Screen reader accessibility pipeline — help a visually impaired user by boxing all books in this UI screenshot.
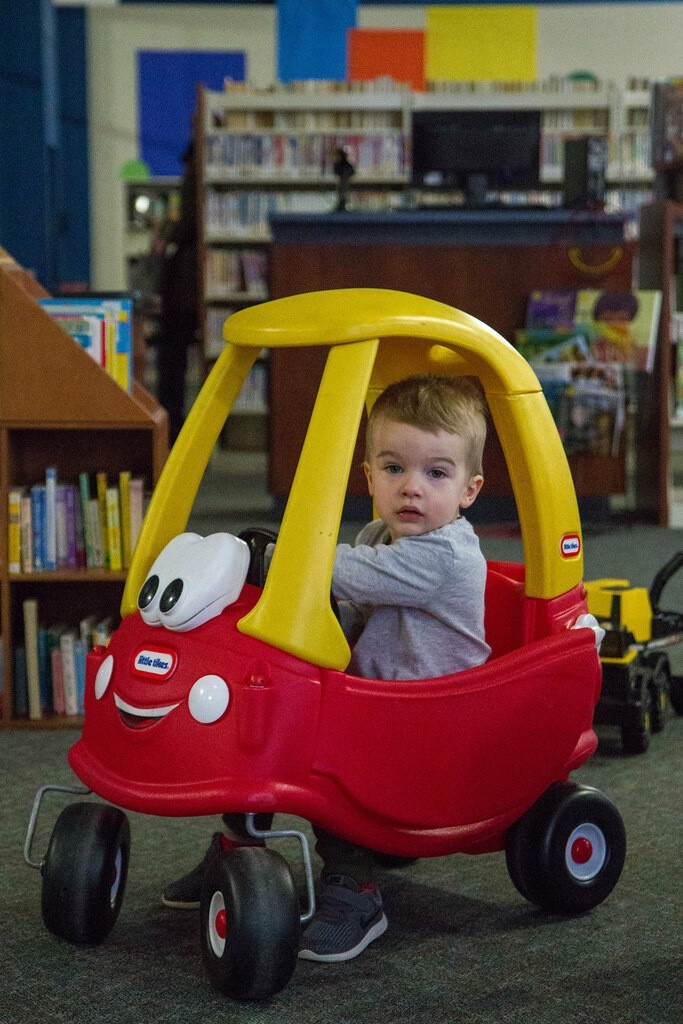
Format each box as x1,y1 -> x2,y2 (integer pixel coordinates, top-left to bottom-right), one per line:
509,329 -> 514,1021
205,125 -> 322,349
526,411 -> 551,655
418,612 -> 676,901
203,76 -> 652,413
38,296 -> 132,396
7,461 -> 151,575
11,597 -> 120,721
512,284 -> 664,458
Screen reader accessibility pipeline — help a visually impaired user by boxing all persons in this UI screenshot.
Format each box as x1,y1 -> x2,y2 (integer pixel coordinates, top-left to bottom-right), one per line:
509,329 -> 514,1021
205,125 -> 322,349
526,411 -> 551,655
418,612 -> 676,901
152,141 -> 201,449
159,374 -> 493,960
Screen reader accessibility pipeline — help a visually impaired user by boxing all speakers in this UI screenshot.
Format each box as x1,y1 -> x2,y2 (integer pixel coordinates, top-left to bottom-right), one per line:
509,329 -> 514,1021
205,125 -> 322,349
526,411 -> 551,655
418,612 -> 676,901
562,134 -> 610,211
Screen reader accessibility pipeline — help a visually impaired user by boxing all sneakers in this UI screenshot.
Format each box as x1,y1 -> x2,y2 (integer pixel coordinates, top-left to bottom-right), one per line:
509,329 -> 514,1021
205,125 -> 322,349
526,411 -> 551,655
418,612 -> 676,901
160,831 -> 271,910
295,863 -> 390,962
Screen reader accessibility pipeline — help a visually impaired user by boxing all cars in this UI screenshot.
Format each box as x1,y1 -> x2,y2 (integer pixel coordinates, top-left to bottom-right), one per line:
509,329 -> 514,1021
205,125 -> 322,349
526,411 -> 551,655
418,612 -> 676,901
37,289 -> 629,1009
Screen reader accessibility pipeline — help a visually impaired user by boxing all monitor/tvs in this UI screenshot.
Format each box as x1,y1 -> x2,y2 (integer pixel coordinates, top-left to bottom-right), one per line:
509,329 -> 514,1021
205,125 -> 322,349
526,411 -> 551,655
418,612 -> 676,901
409,110 -> 540,208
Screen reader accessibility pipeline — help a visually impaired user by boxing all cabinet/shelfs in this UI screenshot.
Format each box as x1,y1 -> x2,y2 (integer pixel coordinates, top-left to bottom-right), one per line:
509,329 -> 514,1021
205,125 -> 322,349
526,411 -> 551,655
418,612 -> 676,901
0,248 -> 168,730
195,82 -> 683,529
